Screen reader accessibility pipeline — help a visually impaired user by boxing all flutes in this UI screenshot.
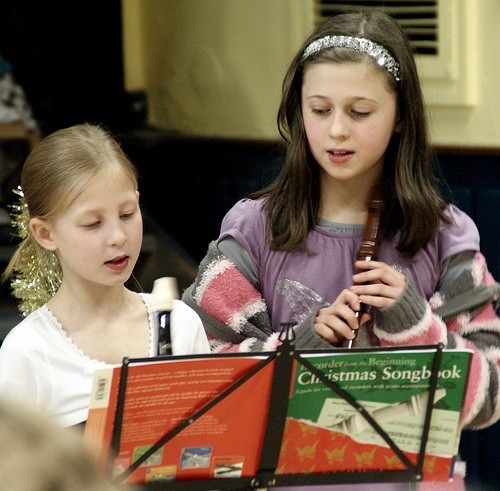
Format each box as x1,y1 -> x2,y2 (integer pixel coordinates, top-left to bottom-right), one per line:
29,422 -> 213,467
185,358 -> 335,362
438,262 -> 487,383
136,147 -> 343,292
340,182 -> 390,350
151,276 -> 182,356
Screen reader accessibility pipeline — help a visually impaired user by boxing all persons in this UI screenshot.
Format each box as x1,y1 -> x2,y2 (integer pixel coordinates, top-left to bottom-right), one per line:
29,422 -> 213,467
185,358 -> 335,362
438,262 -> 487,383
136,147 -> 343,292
1,125 -> 212,447
0,65 -> 43,242
182,10 -> 500,480
